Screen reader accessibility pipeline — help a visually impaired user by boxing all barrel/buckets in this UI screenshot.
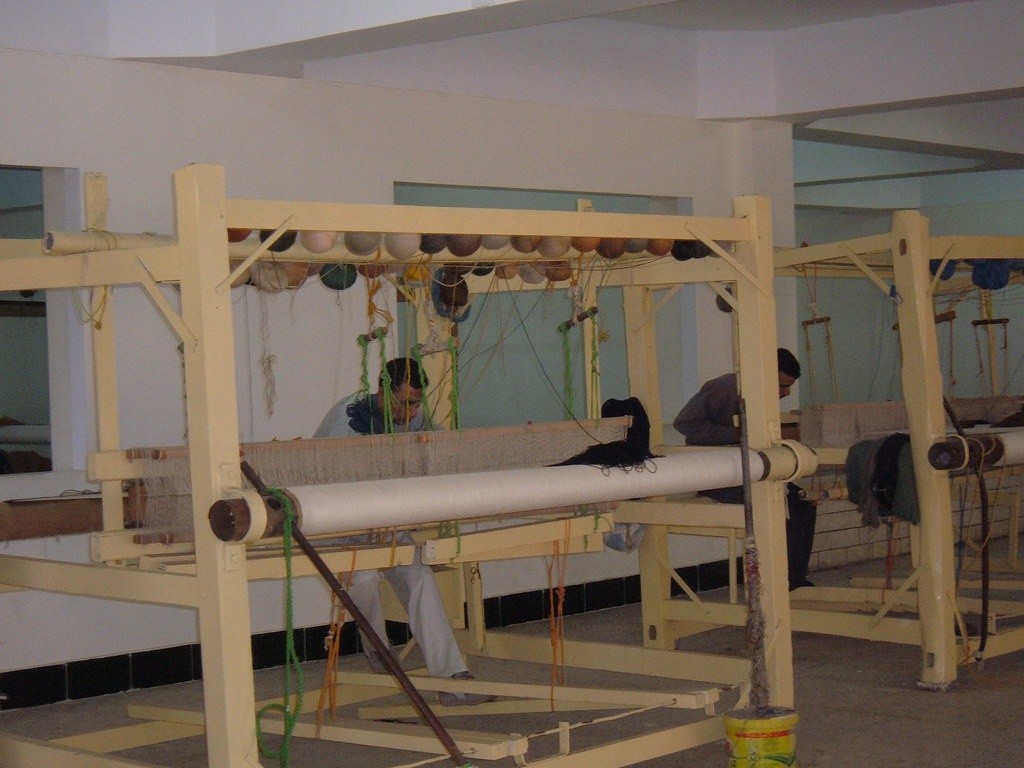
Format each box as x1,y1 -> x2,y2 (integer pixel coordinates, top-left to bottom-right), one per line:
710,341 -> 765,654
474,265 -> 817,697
723,703 -> 801,768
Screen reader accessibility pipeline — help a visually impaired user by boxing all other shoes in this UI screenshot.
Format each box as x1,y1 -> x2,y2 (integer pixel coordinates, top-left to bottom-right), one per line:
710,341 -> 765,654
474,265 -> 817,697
789,577 -> 815,592
361,638 -> 399,675
437,671 -> 498,706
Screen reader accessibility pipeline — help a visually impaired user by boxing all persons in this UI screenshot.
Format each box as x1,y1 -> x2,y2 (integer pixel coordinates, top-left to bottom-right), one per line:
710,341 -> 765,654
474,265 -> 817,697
311,358 -> 496,705
674,348 -> 815,590
